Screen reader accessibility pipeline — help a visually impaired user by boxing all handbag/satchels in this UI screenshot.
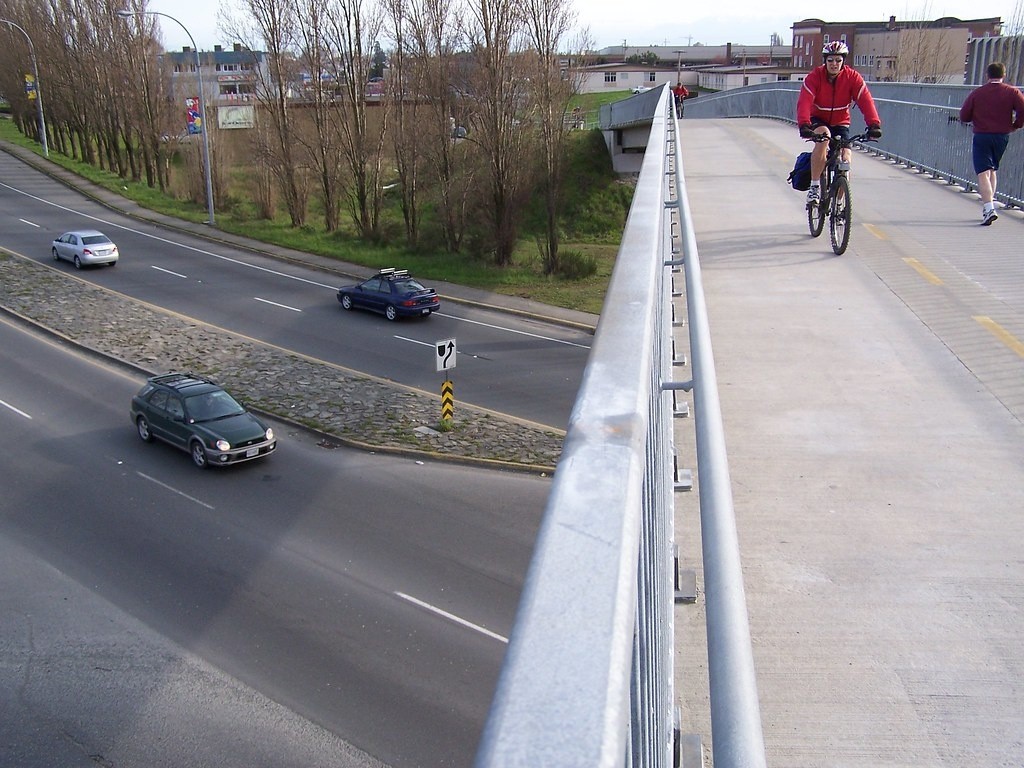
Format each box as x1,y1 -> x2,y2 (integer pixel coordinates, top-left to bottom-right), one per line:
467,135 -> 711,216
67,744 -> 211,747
787,153 -> 815,191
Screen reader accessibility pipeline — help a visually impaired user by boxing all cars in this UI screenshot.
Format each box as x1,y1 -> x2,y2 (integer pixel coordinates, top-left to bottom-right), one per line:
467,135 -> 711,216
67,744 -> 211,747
52,229 -> 119,270
129,372 -> 278,470
336,267 -> 440,321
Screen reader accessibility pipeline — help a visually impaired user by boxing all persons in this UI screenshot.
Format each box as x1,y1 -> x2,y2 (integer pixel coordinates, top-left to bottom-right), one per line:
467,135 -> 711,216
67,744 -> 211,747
206,397 -> 213,406
960,62 -> 1024,226
674,82 -> 689,117
795,40 -> 882,226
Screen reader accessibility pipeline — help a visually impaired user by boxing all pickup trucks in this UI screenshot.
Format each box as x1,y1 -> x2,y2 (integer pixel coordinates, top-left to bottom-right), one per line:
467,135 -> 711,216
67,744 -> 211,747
629,85 -> 655,94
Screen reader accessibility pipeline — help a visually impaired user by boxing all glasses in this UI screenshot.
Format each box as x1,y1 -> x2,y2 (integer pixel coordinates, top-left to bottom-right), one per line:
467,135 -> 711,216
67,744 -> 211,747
826,57 -> 845,62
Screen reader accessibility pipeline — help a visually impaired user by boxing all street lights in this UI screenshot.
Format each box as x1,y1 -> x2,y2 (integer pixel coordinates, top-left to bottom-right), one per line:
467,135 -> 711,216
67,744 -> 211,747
116,10 -> 216,225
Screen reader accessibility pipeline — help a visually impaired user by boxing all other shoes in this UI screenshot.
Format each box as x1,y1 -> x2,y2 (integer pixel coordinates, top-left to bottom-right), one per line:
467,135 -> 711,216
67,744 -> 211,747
981,209 -> 998,225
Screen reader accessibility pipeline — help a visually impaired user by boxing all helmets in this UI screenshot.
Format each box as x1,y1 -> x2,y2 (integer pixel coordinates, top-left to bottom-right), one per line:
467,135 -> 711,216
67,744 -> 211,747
822,40 -> 849,57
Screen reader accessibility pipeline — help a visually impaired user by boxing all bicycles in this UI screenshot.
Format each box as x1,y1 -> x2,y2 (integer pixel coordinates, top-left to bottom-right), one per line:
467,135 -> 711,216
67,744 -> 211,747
800,133 -> 882,255
675,95 -> 687,119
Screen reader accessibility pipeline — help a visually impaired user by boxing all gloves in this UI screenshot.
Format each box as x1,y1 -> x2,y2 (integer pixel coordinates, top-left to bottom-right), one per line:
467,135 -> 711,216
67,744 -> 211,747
800,127 -> 813,138
867,129 -> 881,138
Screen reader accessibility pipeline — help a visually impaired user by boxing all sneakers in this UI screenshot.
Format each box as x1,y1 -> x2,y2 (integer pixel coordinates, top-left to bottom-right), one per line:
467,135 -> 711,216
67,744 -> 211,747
836,208 -> 844,226
807,185 -> 820,204
